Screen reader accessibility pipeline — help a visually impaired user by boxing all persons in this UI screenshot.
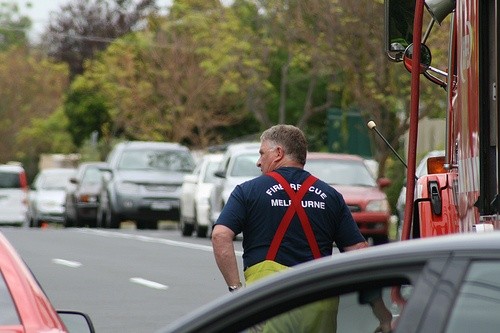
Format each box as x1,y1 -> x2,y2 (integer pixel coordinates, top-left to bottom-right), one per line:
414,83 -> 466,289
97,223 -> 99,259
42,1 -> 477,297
210,124 -> 393,333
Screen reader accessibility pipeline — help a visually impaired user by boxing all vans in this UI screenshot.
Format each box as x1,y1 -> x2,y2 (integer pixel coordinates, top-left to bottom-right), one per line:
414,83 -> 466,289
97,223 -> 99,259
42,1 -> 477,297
0,164 -> 27,226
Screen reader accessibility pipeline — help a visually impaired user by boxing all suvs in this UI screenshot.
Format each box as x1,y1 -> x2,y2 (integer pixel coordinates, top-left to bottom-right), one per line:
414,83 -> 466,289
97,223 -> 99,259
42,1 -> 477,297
97,142 -> 196,228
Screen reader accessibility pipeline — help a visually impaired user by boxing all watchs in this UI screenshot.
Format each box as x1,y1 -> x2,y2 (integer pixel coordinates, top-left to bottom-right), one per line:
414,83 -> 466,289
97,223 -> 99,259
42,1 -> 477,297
228,282 -> 242,292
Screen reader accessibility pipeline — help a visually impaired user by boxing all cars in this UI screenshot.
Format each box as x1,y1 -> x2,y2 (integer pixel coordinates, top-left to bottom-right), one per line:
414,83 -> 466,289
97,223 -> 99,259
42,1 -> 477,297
302,152 -> 392,246
62,161 -> 106,227
26,168 -> 74,229
0,230 -> 95,333
173,151 -> 226,238
207,142 -> 265,240
